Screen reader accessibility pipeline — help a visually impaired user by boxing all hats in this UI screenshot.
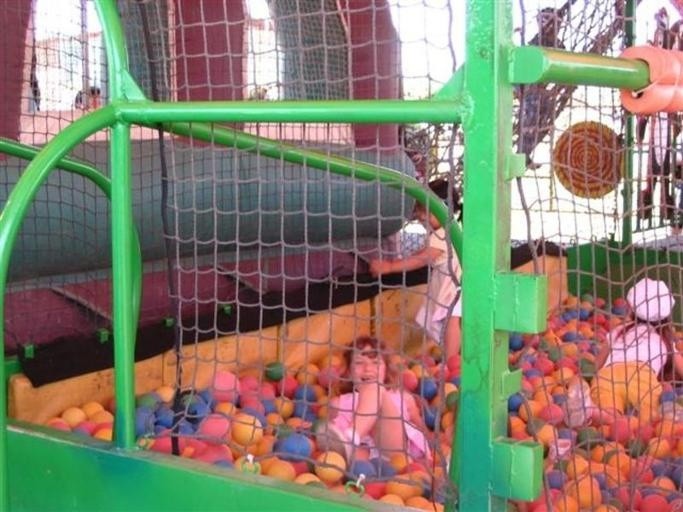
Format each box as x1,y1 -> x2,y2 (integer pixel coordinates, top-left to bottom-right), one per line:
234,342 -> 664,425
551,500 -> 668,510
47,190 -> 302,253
627,278 -> 676,323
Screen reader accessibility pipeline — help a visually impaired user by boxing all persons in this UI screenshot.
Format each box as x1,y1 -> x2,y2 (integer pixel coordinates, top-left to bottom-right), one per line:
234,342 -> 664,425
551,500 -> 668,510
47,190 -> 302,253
369,181 -> 462,370
315,335 -> 432,484
514,9 -> 567,168
590,277 -> 683,422
76,87 -> 100,108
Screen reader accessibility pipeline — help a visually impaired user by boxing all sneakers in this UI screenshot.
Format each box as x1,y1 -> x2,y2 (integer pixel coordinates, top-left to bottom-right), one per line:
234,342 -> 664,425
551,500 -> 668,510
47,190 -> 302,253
317,425 -> 349,465
